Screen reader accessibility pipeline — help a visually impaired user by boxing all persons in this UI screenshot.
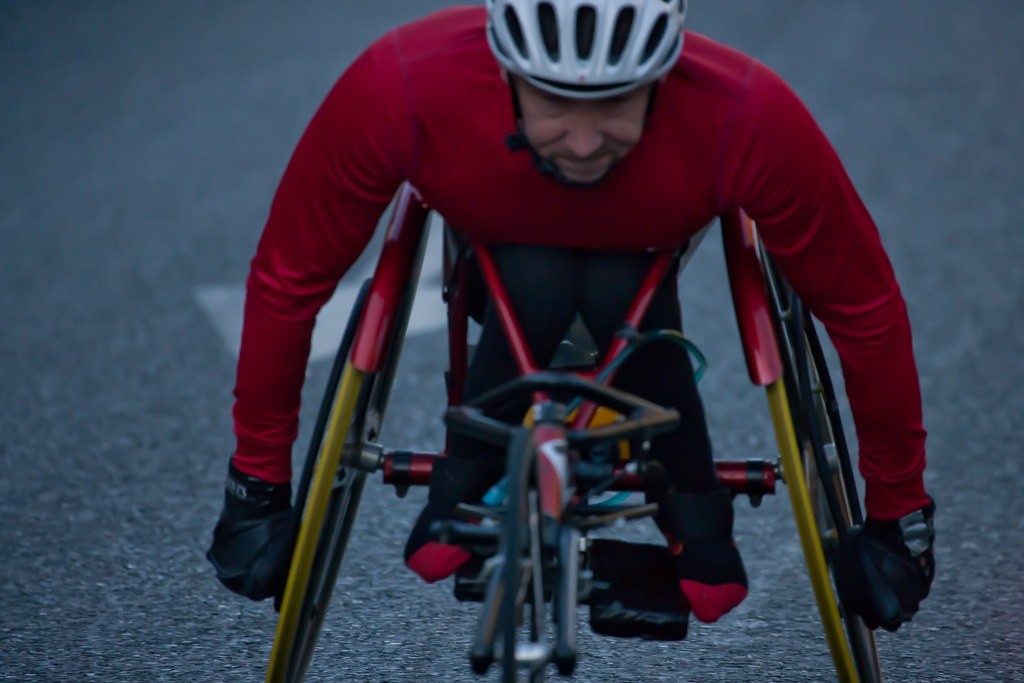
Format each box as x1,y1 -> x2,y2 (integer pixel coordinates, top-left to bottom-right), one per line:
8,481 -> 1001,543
205,0 -> 938,633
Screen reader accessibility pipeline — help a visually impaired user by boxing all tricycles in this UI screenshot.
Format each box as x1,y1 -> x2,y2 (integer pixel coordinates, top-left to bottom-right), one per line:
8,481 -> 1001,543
265,174 -> 881,683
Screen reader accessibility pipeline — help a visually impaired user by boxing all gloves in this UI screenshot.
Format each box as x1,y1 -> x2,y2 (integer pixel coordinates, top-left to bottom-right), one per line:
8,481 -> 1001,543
205,453 -> 296,612
839,493 -> 936,633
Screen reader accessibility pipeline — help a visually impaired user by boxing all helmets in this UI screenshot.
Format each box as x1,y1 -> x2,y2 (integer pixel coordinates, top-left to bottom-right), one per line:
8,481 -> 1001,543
485,0 -> 688,99
